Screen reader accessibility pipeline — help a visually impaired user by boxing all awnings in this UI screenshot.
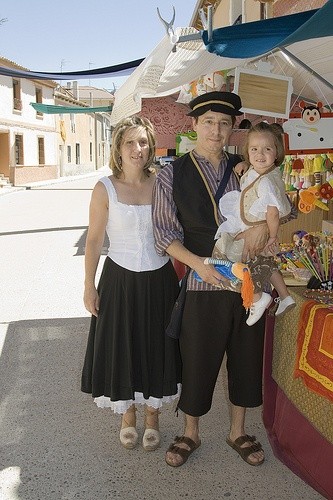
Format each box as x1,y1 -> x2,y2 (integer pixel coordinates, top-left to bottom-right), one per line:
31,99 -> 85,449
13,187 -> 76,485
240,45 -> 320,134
109,0 -> 332,128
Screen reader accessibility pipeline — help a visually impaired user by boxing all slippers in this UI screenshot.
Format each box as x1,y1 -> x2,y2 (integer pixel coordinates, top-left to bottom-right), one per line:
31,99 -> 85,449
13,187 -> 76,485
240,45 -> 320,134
166,436 -> 202,467
226,434 -> 266,466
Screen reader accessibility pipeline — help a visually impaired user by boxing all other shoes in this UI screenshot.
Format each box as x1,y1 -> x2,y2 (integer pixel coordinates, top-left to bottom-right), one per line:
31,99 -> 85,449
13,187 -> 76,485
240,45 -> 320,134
274,295 -> 295,317
246,291 -> 273,326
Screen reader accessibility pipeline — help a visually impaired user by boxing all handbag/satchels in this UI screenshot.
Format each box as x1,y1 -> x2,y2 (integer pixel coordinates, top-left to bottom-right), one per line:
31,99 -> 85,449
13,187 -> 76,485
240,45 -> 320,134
166,272 -> 187,341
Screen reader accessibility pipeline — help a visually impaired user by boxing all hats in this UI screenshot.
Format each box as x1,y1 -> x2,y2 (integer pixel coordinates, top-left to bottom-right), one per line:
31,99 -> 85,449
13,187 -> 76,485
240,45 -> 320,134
185,90 -> 245,117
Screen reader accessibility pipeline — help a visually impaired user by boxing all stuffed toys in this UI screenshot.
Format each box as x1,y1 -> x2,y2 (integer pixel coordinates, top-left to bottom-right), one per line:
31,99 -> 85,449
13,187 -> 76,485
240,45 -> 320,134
192,257 -> 256,309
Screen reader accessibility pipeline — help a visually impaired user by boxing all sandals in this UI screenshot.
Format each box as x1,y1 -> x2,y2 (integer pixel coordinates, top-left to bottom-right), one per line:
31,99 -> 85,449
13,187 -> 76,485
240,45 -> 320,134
143,410 -> 161,450
120,412 -> 138,448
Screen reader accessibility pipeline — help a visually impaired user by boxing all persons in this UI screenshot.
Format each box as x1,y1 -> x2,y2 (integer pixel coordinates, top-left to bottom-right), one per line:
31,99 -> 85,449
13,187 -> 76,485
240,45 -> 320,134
82,114 -> 184,453
149,89 -> 272,468
208,120 -> 297,328
